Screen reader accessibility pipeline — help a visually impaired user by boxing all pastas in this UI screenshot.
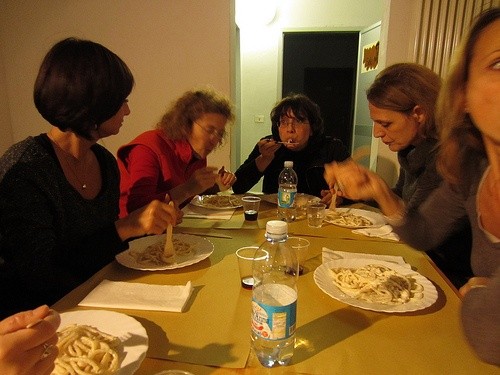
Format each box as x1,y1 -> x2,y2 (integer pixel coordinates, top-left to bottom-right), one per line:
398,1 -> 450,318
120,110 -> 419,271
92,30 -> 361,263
51,322 -> 123,375
327,264 -> 424,306
129,237 -> 192,264
324,212 -> 371,227
201,195 -> 243,207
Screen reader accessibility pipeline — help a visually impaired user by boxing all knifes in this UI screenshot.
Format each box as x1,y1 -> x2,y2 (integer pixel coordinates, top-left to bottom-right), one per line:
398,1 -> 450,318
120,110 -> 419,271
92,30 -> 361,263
177,232 -> 233,239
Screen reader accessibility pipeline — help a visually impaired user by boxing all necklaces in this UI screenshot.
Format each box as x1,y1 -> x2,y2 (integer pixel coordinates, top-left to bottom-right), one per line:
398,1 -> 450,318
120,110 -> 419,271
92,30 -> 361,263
56,139 -> 87,189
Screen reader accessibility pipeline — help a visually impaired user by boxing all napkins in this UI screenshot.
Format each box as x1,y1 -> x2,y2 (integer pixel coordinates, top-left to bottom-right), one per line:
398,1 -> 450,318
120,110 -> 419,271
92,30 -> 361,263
352,225 -> 401,242
322,246 -> 412,270
77,279 -> 194,312
182,206 -> 236,219
176,219 -> 216,228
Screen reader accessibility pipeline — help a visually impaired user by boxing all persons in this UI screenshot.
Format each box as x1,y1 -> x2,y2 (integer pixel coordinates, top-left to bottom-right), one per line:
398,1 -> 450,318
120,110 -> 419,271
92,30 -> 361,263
324,6 -> 500,366
0,38 -> 184,375
234,94 -> 352,209
115,87 -> 236,230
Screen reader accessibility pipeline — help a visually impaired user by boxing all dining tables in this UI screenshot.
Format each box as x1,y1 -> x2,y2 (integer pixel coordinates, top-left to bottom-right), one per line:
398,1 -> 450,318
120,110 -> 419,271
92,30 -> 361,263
51,194 -> 500,375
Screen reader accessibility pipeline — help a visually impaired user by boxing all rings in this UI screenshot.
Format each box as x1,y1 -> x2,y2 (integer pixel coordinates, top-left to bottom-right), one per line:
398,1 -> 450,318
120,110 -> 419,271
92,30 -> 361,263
41,343 -> 54,358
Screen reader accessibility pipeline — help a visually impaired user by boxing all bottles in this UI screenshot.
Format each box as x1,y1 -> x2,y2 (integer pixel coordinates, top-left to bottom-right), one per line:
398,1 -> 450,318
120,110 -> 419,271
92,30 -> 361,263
277,161 -> 298,224
251,220 -> 299,369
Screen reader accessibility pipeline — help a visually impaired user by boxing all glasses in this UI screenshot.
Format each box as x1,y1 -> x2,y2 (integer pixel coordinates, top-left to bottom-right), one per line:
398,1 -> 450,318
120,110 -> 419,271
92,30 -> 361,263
193,121 -> 226,140
276,115 -> 305,128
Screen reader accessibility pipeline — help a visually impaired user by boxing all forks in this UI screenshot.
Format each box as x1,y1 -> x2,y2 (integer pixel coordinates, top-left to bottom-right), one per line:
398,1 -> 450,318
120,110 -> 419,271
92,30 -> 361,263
164,200 -> 174,257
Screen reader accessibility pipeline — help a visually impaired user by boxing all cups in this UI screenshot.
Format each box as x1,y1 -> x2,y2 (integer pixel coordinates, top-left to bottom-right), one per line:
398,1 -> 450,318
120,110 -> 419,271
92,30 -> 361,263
241,196 -> 261,221
306,203 -> 327,229
235,247 -> 269,290
285,237 -> 310,276
154,370 -> 194,375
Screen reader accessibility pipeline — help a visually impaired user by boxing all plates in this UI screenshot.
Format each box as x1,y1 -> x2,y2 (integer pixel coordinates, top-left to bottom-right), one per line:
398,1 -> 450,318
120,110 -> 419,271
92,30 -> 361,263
115,234 -> 213,271
49,310 -> 149,375
312,259 -> 438,313
322,209 -> 388,228
190,195 -> 244,209
263,193 -> 321,206
